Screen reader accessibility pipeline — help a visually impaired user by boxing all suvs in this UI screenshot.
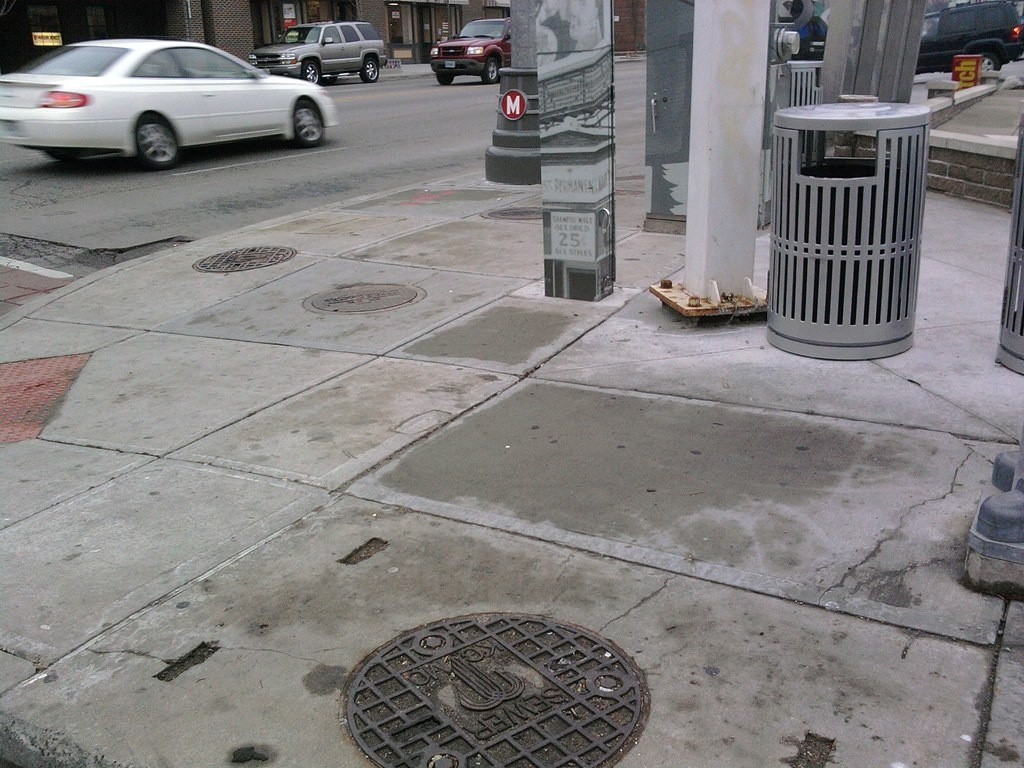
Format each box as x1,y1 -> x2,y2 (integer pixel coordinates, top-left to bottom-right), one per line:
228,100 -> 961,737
247,22 -> 390,85
428,16 -> 512,86
913,1 -> 1024,77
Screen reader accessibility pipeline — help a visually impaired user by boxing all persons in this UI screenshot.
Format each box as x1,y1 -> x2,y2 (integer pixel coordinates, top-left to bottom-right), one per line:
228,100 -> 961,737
790,0 -> 828,59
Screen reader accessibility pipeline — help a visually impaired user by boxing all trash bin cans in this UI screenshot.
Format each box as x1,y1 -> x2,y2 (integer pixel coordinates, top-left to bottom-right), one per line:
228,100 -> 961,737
787,61 -> 820,163
996,111 -> 1024,376
767,101 -> 929,360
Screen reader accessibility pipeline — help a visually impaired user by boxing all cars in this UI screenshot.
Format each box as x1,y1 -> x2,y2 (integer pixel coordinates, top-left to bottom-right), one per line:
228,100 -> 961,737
0,37 -> 341,174
